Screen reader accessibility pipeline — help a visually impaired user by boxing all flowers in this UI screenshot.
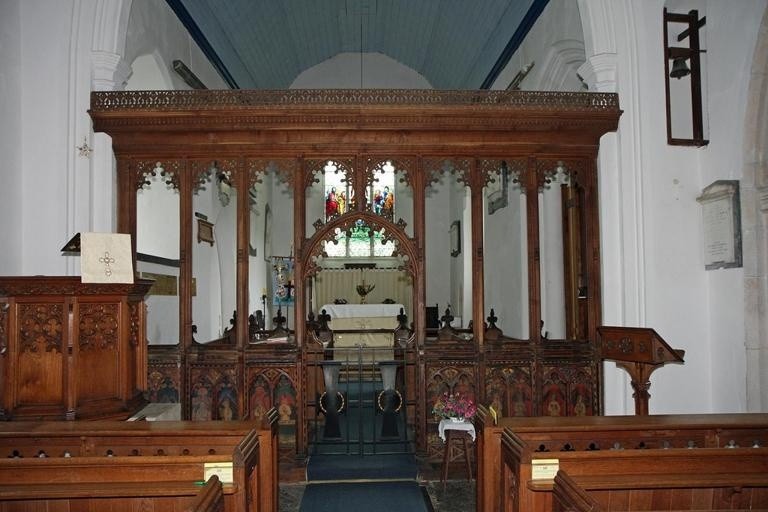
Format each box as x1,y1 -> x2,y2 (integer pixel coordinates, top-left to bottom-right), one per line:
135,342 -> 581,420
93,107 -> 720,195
432,391 -> 477,419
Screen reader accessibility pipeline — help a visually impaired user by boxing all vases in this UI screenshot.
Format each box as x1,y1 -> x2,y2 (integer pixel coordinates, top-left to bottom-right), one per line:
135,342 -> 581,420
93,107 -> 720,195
448,413 -> 466,422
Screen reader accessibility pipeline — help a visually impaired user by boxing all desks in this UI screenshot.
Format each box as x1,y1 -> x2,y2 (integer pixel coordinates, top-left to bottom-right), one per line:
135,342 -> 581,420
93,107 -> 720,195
438,419 -> 477,486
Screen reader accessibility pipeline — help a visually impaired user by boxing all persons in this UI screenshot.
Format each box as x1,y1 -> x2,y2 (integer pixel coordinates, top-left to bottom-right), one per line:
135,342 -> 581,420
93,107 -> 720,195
327,186 -> 393,226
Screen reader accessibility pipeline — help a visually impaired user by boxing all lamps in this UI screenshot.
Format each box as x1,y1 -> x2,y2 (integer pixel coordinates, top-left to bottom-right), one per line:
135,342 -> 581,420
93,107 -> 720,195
661,7 -> 709,149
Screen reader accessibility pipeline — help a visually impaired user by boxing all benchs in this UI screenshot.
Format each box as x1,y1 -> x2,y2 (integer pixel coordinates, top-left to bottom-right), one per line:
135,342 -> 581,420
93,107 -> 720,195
476,411 -> 731,509
0,405 -> 280,511
